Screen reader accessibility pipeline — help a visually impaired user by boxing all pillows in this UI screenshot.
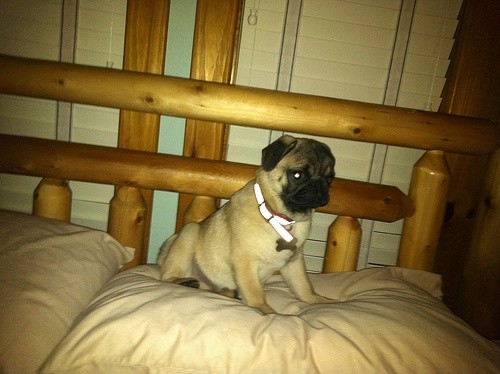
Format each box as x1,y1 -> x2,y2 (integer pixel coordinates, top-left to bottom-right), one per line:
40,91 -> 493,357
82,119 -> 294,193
0,208 -> 136,374
36,264 -> 500,374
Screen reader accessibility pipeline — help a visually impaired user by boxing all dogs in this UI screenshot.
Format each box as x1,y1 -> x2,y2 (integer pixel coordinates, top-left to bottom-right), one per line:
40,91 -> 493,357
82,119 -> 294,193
155,134 -> 338,319
